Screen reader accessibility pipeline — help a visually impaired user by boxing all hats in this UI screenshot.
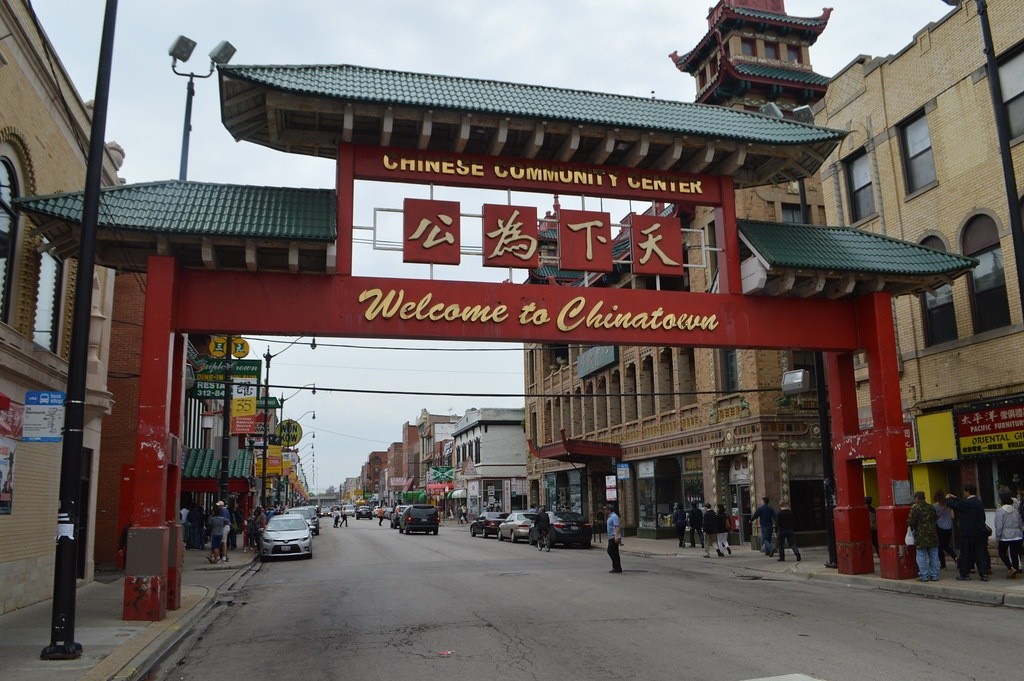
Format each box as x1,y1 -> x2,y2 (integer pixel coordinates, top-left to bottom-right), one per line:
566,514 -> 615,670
604,504 -> 614,510
216,500 -> 224,506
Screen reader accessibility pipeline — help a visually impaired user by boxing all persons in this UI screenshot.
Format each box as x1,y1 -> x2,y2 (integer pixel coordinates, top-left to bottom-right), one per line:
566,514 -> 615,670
533,506 -> 551,541
334,509 -> 339,528
906,492 -> 940,581
557,502 -> 571,512
996,479 -> 1024,580
486,505 -> 502,512
457,503 -> 468,524
866,496 -> 879,557
931,489 -> 960,569
339,511 -> 347,527
118,523 -> 131,570
672,501 -> 686,548
378,505 -> 385,526
689,500 -> 732,558
602,504 -> 622,573
749,496 -> 776,558
206,500 -> 243,563
945,485 -> 991,582
180,501 -> 205,551
671,501 -> 679,514
529,502 -> 538,513
250,500 -> 291,551
774,497 -> 802,561
448,506 -> 456,522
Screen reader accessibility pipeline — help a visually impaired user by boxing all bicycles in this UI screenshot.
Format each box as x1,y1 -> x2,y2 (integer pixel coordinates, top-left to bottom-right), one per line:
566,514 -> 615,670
536,528 -> 551,552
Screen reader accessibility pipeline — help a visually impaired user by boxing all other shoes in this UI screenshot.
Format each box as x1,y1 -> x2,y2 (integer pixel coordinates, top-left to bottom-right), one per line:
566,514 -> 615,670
1014,573 -> 1019,579
679,540 -> 685,547
716,549 -> 724,557
609,568 -> 622,573
689,544 -> 695,547
766,550 -> 774,557
702,542 -> 704,548
778,558 -> 785,561
1006,567 -> 1016,578
703,555 -> 710,558
955,556 -> 961,569
797,553 -> 801,561
939,566 -> 947,571
956,574 -> 971,580
981,574 -> 988,580
726,546 -> 731,554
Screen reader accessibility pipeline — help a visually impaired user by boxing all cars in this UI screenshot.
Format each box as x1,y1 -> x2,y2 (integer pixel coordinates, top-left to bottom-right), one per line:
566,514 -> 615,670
319,504 -> 332,516
497,512 -> 538,542
356,506 -> 372,520
470,512 -> 509,538
342,504 -> 355,517
372,506 -> 394,519
282,505 -> 320,535
528,511 -> 592,548
259,514 -> 317,560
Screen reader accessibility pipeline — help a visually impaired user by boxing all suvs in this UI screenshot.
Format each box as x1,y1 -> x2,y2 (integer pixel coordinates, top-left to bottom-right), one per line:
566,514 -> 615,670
398,504 -> 439,535
390,505 -> 409,529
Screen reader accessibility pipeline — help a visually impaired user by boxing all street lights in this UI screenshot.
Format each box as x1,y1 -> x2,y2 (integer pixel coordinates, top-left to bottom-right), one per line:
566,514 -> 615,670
291,432 -> 315,507
277,382 -> 316,507
169,35 -> 236,182
285,410 -> 315,507
261,336 -> 317,508
757,101 -> 840,568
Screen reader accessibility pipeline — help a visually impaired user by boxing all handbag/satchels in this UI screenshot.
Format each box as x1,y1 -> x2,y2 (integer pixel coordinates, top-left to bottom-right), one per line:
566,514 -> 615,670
985,524 -> 992,536
905,526 -> 914,545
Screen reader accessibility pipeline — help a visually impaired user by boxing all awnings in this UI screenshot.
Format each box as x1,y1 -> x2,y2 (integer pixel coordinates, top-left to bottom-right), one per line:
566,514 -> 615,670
446,489 -> 466,498
402,490 -> 427,500
